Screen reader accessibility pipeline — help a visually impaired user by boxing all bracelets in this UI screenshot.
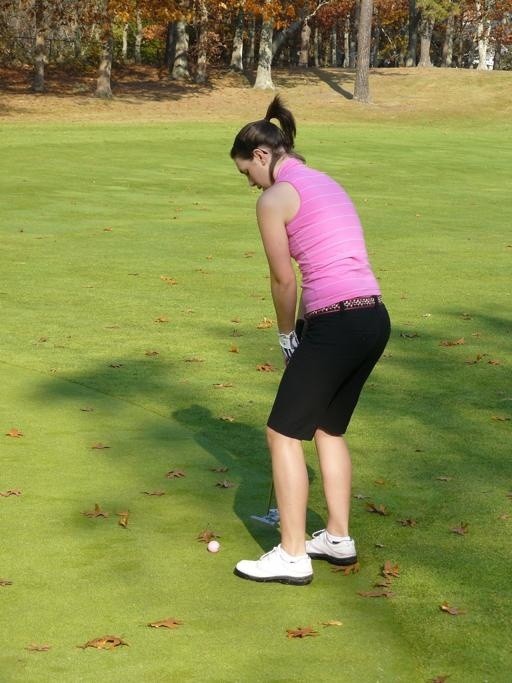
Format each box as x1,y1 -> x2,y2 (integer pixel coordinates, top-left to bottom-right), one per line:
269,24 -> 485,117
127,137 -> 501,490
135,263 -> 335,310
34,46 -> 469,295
277,329 -> 299,359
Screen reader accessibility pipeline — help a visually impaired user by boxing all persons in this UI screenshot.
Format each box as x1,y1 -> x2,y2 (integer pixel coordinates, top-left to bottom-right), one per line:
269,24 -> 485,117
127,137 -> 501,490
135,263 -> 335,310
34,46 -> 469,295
229,95 -> 391,584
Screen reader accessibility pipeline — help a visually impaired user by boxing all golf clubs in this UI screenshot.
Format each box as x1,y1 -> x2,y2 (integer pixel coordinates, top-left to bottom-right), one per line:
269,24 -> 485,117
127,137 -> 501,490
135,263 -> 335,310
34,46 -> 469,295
251,317 -> 306,526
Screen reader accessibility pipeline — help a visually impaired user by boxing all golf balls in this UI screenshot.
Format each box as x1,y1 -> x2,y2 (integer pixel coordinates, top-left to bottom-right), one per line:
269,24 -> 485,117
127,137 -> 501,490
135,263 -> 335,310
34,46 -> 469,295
209,537 -> 223,556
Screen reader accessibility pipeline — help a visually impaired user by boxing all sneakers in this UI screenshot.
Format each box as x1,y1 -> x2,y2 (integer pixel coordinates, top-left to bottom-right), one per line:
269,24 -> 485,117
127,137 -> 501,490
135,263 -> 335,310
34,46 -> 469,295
235,543 -> 314,586
305,527 -> 358,567
